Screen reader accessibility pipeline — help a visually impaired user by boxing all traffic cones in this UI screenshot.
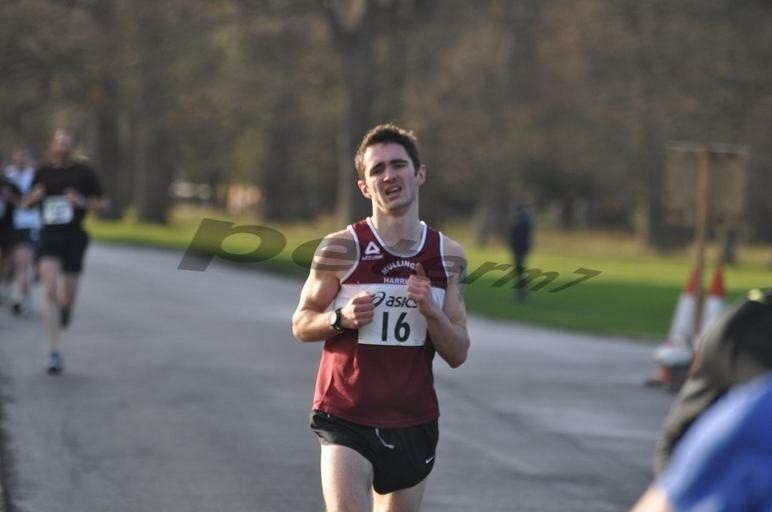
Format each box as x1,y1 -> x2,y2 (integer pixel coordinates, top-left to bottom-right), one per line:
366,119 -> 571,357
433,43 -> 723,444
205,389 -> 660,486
660,266 -> 724,380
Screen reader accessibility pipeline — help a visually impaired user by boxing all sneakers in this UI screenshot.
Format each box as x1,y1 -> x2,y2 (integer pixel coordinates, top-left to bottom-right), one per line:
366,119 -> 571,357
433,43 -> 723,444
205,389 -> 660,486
45,304 -> 70,374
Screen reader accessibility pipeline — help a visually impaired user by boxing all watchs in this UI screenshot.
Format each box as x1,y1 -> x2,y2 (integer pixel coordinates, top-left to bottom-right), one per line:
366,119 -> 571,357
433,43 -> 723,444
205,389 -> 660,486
329,308 -> 345,334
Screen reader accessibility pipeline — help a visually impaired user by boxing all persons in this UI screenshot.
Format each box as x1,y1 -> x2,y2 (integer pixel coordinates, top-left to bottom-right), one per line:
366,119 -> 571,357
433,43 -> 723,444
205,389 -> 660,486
620,285 -> 772,512
511,197 -> 535,294
0,128 -> 114,375
292,124 -> 469,512
